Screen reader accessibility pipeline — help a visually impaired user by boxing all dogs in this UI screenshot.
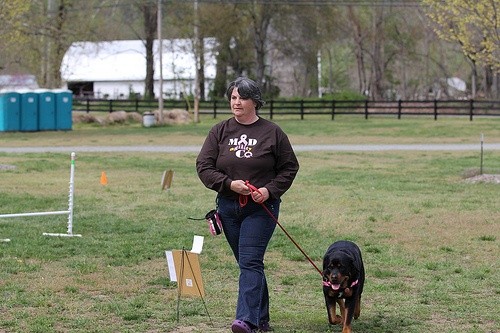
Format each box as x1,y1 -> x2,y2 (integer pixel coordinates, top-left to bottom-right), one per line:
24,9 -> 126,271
322,240 -> 365,333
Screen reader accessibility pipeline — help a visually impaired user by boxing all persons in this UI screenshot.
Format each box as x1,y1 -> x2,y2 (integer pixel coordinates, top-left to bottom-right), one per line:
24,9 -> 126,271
195,76 -> 301,333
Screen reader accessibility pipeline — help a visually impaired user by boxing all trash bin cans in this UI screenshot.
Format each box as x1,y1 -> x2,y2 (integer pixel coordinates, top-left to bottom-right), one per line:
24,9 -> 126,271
0,90 -> 20,133
36,89 -> 56,131
53,89 -> 73,132
19,89 -> 37,132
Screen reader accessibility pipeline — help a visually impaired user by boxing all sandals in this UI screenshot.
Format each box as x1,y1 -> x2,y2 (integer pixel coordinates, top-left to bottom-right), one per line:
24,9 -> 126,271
259,323 -> 273,331
231,320 -> 251,333
